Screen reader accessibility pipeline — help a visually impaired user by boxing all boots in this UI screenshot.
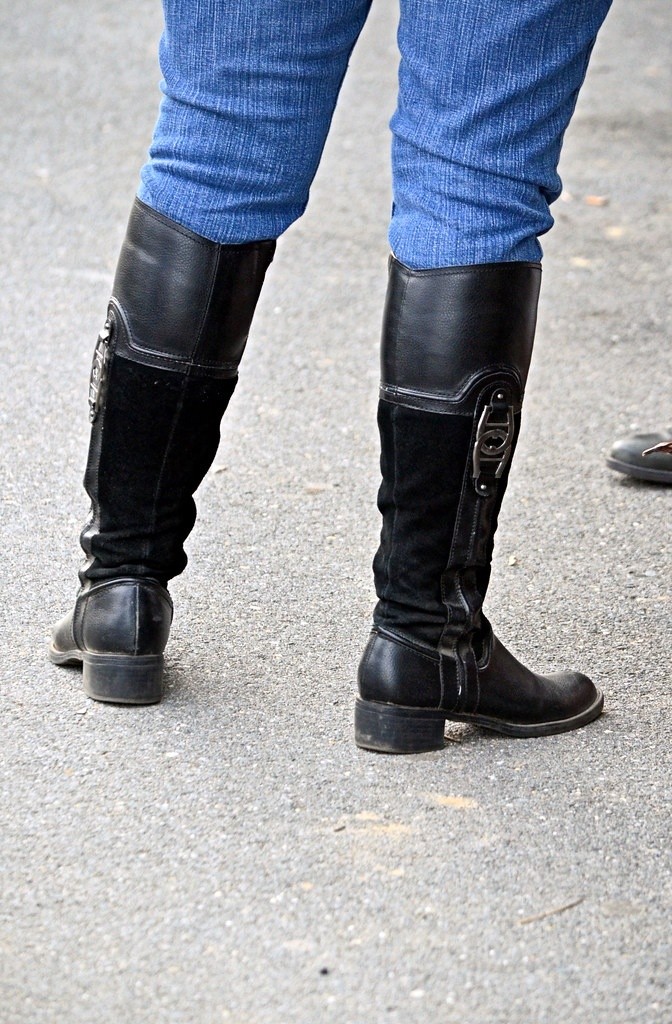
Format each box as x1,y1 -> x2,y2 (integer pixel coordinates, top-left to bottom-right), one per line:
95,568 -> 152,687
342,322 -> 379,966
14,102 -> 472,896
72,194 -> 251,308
354,251 -> 604,756
49,198 -> 277,706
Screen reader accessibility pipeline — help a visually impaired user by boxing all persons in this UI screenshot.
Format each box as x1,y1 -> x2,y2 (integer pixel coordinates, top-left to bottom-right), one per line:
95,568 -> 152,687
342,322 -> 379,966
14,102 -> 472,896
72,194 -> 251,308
49,0 -> 604,753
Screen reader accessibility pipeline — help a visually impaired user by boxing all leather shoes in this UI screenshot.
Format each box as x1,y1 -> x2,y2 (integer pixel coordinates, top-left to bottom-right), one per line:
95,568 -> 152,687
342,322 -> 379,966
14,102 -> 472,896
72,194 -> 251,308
606,431 -> 670,483
